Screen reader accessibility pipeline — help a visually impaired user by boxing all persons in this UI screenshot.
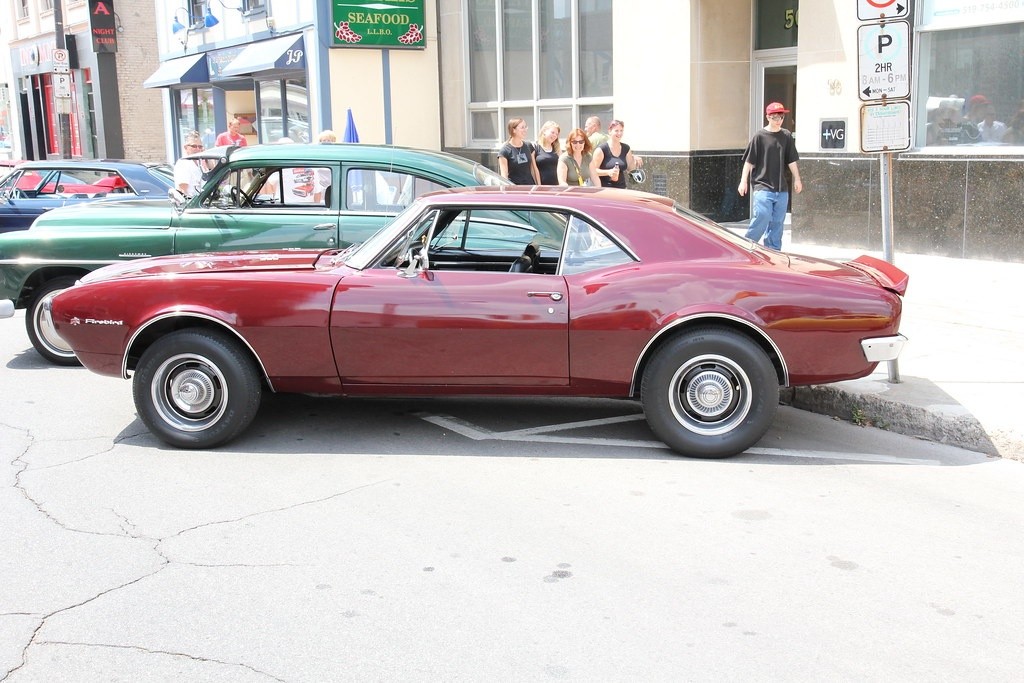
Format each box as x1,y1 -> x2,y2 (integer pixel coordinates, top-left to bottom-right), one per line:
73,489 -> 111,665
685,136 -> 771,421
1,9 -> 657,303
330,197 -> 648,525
555,128 -> 602,188
592,120 -> 643,190
582,116 -> 610,159
214,117 -> 248,189
173,130 -> 222,200
530,121 -> 563,186
258,137 -> 321,204
738,102 -> 802,251
926,92 -> 1024,147
312,129 -> 347,204
496,117 -> 538,185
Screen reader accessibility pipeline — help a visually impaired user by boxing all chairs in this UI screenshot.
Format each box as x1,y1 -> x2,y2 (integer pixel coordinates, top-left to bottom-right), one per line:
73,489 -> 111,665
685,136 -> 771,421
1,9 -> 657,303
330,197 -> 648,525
325,185 -> 353,208
508,242 -> 541,273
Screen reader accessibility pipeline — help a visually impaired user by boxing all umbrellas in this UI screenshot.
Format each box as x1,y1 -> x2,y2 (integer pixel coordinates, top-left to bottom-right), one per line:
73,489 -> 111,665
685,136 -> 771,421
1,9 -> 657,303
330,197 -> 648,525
341,106 -> 367,203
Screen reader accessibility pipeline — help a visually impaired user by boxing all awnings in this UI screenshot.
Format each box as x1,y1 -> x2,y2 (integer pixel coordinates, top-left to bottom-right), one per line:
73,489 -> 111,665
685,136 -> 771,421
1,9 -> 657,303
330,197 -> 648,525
221,32 -> 305,79
142,51 -> 210,89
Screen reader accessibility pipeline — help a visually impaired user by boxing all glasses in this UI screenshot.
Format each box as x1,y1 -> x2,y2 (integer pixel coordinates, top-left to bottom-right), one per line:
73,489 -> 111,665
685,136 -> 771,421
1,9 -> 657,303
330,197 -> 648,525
187,144 -> 203,149
516,126 -> 528,130
769,115 -> 784,121
570,139 -> 584,144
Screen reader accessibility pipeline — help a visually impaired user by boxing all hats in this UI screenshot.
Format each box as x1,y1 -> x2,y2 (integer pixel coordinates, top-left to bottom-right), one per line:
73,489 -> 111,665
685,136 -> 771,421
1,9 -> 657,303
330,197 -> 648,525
968,95 -> 995,110
766,102 -> 790,115
627,168 -> 646,185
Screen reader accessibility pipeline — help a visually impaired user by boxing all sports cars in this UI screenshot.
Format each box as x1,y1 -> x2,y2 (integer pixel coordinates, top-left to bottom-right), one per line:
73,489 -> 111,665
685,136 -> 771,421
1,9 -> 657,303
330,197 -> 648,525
47,183 -> 910,460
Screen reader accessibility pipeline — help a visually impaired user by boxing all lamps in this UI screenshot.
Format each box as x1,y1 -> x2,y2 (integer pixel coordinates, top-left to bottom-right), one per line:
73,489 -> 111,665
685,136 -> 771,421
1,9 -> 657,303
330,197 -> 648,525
173,7 -> 205,34
205,0 -> 241,27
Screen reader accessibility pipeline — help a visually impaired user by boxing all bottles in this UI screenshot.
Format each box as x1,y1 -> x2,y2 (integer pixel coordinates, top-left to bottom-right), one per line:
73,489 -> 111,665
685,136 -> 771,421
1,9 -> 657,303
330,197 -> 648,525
611,163 -> 619,181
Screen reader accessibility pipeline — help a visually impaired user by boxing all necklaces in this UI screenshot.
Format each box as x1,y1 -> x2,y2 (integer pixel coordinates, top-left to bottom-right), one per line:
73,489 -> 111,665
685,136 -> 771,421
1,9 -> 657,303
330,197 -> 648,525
513,143 -> 522,155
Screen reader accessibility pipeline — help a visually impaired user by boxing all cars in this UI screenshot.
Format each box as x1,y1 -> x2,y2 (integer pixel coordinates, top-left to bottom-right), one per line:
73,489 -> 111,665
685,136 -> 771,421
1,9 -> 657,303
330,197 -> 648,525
0,158 -> 275,237
0,140 -> 606,368
